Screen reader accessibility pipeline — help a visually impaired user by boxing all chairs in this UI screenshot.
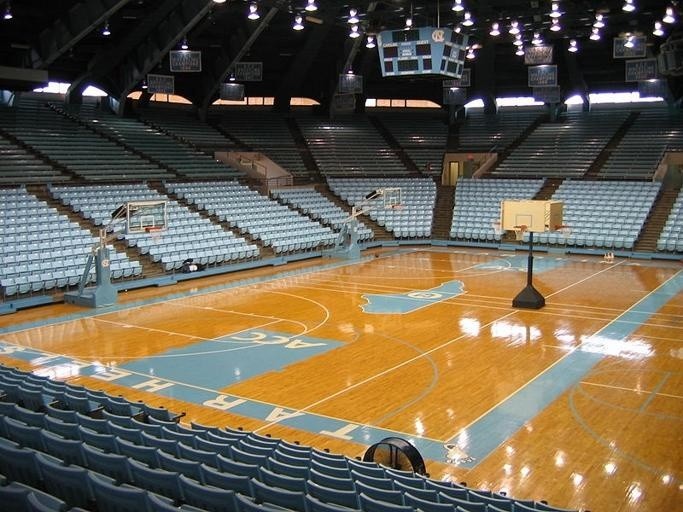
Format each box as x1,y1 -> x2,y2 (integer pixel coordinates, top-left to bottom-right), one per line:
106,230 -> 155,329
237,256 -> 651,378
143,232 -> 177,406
0,352 -> 586,512
0,92 -> 682,300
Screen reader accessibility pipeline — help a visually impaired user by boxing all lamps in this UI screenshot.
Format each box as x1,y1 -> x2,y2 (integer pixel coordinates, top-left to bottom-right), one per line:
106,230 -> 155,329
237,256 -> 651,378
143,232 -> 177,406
292,14 -> 304,30
341,1 -> 682,91
247,3 -> 260,20
229,70 -> 235,81
102,20 -> 111,35
142,77 -> 148,89
3,5 -> 13,20
181,35 -> 188,49
305,1 -> 318,12
